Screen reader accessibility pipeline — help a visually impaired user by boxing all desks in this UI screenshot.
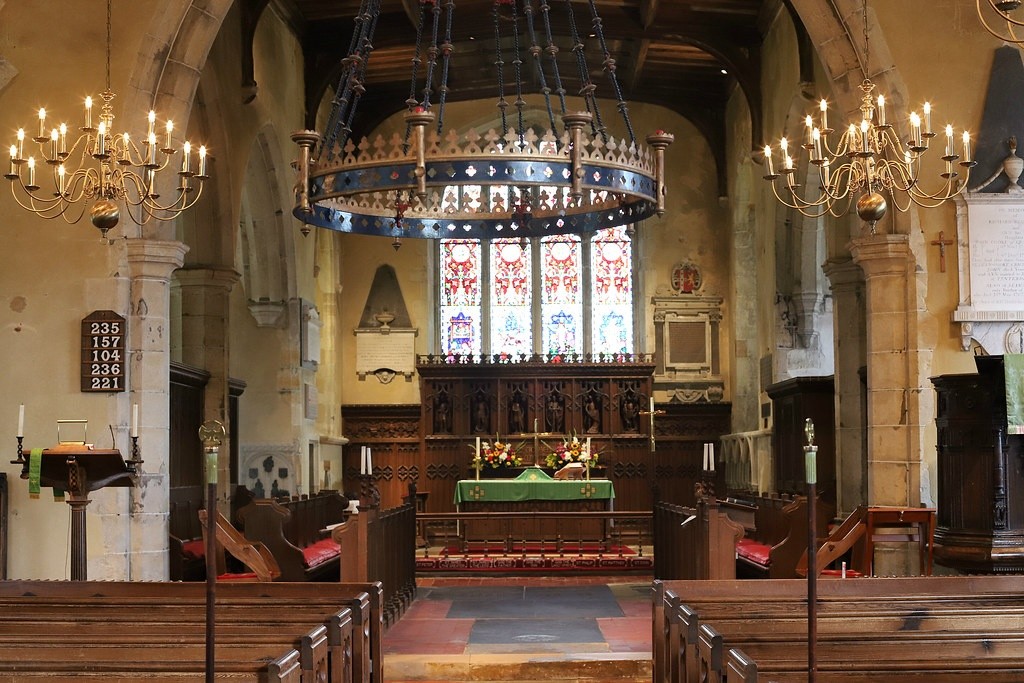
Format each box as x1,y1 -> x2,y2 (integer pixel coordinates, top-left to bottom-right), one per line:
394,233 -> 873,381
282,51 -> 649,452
454,477 -> 616,541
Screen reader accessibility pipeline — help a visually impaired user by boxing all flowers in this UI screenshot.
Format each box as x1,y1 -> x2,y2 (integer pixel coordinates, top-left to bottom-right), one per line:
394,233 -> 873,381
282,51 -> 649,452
540,426 -> 615,471
466,431 -> 528,471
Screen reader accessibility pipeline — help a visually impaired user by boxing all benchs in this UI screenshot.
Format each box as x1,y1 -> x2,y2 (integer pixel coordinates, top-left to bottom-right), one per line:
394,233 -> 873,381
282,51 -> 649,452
736,492 -> 829,579
0,577 -> 386,682
169,534 -> 206,581
243,487 -> 350,581
651,574 -> 1024,683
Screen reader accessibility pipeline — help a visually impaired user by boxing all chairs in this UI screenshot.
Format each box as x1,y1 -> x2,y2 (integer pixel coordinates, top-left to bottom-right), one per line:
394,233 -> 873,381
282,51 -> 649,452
198,509 -> 281,582
795,506 -> 867,578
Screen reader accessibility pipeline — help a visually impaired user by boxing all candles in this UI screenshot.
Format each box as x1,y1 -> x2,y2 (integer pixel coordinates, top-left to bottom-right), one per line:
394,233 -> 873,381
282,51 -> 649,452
475,436 -> 481,457
132,402 -> 138,435
586,436 -> 591,457
361,445 -> 366,474
703,444 -> 715,470
366,447 -> 373,475
650,396 -> 655,412
17,402 -> 25,436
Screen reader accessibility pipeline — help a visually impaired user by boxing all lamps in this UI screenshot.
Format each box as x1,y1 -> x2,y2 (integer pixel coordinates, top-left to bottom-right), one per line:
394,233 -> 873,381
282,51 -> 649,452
3,0 -> 209,246
761,0 -> 1024,237
290,1 -> 676,252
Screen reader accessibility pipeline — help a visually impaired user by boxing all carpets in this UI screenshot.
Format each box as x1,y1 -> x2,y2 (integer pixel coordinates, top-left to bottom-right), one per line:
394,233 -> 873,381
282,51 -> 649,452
441,546 -> 638,555
